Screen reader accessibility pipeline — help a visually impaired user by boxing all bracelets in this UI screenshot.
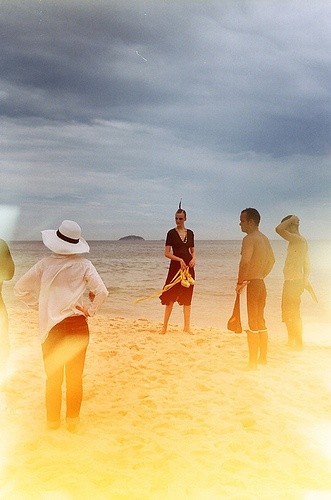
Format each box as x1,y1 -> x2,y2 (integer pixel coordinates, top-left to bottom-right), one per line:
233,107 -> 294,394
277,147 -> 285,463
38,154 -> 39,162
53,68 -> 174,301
237,282 -> 242,285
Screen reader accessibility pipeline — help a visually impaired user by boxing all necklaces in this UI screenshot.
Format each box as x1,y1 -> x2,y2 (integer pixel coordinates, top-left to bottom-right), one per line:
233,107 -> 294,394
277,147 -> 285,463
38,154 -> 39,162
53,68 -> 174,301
174,226 -> 188,244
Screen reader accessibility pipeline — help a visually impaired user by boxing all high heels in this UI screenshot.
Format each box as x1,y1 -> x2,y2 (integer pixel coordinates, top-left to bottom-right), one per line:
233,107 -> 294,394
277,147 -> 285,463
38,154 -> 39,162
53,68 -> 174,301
179,266 -> 196,288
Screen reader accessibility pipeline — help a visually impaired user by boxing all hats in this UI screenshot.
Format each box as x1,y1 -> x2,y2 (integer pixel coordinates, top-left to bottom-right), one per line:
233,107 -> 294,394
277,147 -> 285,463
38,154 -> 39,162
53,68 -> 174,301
41,220 -> 90,255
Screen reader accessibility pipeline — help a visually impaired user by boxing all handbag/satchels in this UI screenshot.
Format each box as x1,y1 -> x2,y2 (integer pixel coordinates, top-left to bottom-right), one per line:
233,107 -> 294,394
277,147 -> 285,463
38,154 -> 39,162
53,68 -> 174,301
227,293 -> 242,333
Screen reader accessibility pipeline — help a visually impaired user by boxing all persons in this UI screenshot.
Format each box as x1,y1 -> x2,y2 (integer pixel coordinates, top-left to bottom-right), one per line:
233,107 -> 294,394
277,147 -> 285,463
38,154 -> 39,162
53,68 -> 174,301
275,215 -> 318,350
15,220 -> 110,431
235,207 -> 276,370
159,202 -> 197,335
0,239 -> 15,356
